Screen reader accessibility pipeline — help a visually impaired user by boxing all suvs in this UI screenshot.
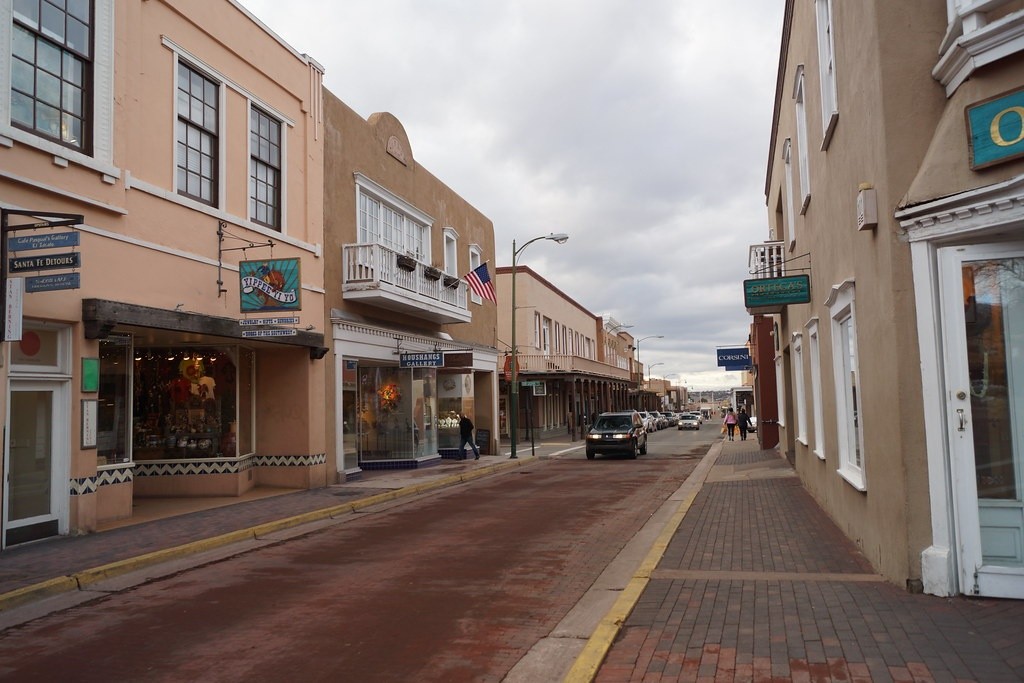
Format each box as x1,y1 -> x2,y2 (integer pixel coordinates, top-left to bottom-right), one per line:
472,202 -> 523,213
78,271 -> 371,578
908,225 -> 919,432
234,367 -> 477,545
586,409 -> 648,459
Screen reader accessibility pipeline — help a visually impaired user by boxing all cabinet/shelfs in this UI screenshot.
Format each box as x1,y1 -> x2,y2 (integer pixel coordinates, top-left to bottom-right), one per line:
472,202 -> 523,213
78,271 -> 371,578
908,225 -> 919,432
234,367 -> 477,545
134,393 -> 221,458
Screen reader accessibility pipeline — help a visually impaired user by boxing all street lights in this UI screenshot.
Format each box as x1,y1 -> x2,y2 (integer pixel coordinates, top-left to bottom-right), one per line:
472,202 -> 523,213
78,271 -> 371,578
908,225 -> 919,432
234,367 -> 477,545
648,363 -> 664,410
637,335 -> 664,411
510,233 -> 569,459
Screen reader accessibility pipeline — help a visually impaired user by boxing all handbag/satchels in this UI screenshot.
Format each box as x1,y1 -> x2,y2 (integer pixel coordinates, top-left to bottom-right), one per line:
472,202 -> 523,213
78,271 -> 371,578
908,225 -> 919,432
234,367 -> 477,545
734,426 -> 739,435
721,424 -> 727,434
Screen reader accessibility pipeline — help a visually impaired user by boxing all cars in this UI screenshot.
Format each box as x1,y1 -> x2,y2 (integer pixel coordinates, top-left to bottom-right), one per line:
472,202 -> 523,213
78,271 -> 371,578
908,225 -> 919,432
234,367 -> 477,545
638,411 -> 689,433
678,411 -> 703,430
747,416 -> 757,433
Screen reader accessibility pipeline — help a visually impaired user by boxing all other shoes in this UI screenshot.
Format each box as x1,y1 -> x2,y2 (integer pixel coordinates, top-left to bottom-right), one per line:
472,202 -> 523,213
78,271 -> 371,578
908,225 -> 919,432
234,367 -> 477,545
475,455 -> 480,460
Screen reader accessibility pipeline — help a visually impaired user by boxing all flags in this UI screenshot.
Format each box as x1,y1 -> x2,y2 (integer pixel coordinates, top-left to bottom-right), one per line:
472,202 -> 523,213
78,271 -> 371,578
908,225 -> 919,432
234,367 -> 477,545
464,261 -> 497,306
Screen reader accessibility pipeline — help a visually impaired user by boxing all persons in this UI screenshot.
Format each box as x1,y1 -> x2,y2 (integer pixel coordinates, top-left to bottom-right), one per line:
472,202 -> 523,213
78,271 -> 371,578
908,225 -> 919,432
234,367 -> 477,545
458,411 -> 479,461
723,407 -> 753,442
